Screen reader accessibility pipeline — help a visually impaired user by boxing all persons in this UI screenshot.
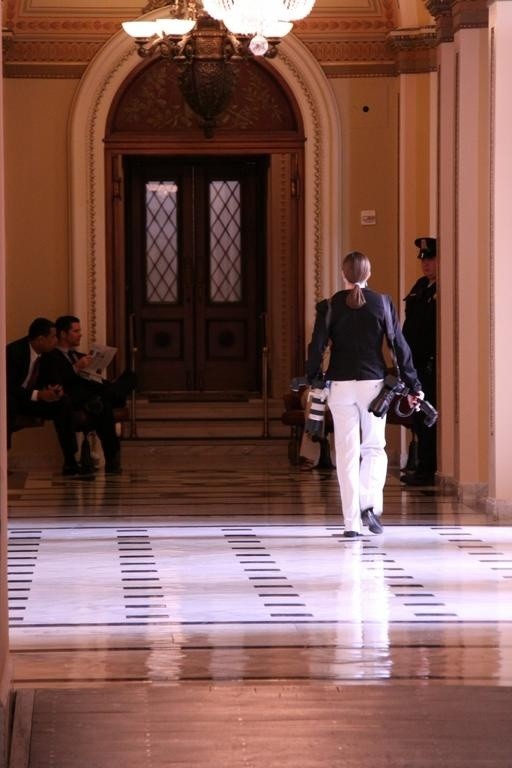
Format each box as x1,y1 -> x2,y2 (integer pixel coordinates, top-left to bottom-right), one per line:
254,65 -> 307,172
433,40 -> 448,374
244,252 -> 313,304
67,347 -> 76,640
5,317 -> 82,474
398,237 -> 437,486
304,251 -> 424,538
42,315 -> 128,473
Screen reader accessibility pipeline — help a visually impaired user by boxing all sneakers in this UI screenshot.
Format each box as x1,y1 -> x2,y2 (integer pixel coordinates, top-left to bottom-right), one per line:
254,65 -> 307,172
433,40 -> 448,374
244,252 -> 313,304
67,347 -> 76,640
399,473 -> 435,486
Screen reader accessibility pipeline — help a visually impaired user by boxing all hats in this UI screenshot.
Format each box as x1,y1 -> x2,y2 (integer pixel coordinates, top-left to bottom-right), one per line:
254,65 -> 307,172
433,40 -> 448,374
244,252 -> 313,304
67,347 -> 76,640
415,238 -> 436,259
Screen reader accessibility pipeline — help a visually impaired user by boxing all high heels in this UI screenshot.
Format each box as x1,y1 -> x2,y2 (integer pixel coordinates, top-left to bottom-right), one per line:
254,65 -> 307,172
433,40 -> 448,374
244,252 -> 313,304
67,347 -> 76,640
344,531 -> 363,537
361,507 -> 384,534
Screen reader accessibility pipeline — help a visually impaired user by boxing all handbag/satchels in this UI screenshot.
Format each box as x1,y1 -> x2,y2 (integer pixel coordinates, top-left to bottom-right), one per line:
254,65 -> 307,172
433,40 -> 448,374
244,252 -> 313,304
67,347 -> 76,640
305,392 -> 327,437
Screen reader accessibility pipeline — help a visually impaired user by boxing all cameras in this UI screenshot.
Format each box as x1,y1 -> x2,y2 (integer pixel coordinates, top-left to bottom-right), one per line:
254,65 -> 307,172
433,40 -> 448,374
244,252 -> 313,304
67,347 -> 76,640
368,378 -> 405,419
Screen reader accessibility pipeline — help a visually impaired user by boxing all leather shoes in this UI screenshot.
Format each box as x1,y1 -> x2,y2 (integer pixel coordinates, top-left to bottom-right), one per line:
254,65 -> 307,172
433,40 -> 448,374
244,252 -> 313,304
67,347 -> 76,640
62,462 -> 93,475
299,458 -> 317,470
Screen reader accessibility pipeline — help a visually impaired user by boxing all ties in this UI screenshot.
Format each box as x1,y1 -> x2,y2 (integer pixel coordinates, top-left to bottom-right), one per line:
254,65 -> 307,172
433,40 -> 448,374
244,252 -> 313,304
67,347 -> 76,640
67,350 -> 77,364
27,358 -> 40,388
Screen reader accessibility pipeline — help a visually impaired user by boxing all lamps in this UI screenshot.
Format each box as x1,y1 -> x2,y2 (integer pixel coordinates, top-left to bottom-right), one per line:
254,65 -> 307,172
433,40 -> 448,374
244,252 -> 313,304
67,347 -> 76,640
119,0 -> 318,140
